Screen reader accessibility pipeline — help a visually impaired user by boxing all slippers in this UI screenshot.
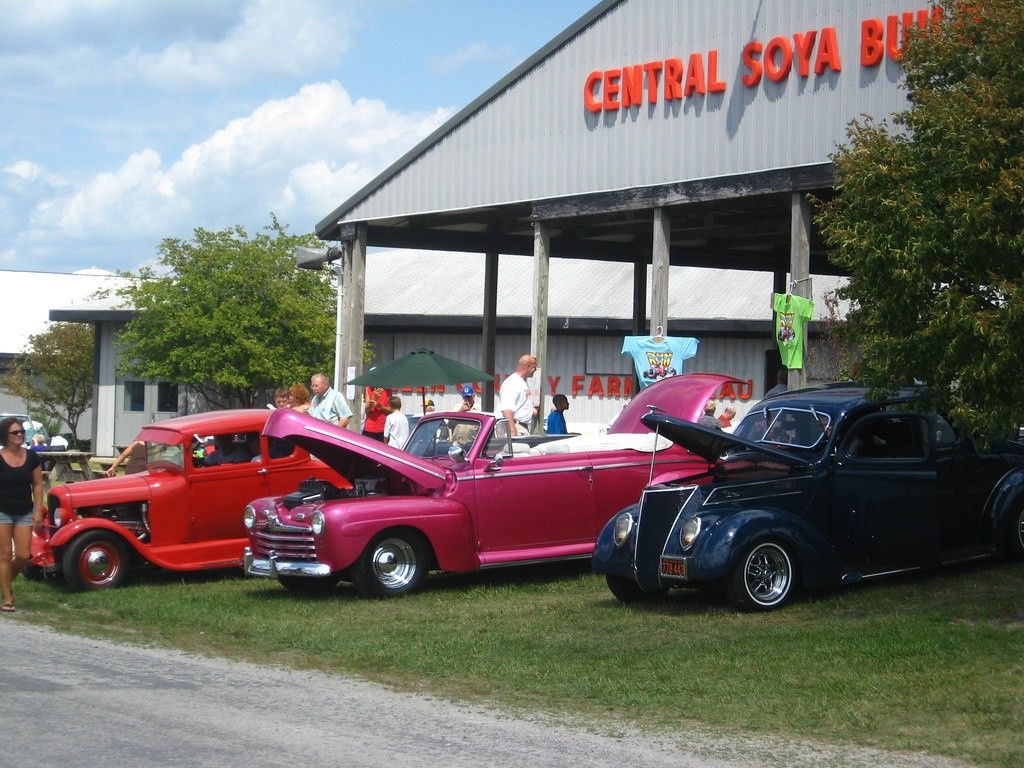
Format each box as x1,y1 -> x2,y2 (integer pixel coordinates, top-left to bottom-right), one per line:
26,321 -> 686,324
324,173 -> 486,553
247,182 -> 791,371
0,604 -> 15,612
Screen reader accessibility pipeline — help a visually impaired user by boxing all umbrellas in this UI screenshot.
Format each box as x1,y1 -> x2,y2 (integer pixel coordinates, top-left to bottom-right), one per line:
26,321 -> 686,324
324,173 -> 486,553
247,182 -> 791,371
343,347 -> 501,416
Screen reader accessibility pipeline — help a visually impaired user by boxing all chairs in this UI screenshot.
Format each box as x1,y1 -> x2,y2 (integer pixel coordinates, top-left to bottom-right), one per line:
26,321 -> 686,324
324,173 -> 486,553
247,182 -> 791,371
499,443 -> 531,459
543,444 -> 571,454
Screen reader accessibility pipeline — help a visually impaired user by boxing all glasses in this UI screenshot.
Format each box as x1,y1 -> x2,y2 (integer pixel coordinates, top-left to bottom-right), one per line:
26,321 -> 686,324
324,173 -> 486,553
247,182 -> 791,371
215,447 -> 226,451
8,430 -> 25,435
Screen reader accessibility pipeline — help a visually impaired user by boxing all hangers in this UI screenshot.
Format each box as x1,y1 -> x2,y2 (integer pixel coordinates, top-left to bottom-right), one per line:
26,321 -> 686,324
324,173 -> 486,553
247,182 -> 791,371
654,326 -> 664,340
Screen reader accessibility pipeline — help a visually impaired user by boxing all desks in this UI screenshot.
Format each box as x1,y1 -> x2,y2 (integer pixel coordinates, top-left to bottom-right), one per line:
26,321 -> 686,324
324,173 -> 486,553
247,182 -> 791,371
112,445 -> 132,472
35,450 -> 97,492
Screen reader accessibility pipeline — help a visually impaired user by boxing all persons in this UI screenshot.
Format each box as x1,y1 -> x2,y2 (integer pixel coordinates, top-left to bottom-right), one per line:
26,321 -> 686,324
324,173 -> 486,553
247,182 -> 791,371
106,439 -> 148,478
452,386 -> 482,412
362,367 -> 392,443
700,400 -> 722,430
499,355 -> 538,436
308,373 -> 350,431
274,387 -> 290,409
0,418 -> 44,611
547,394 -> 569,434
383,396 -> 410,451
821,416 -> 834,435
719,407 -> 736,428
286,384 -> 310,415
424,399 -> 435,412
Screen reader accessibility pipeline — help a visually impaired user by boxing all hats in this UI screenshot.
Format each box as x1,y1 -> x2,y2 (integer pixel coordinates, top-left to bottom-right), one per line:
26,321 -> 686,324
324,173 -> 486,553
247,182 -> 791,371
462,385 -> 475,397
421,400 -> 434,406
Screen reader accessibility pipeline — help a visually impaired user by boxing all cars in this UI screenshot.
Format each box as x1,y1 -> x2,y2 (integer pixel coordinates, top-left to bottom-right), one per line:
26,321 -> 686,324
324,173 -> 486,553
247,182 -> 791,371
0,413 -> 48,449
244,373 -> 748,601
26,405 -> 356,591
592,381 -> 1024,612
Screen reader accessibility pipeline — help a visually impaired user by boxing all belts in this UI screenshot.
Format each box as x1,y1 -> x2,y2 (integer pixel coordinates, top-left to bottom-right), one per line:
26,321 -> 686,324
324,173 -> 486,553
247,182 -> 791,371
515,422 -> 527,427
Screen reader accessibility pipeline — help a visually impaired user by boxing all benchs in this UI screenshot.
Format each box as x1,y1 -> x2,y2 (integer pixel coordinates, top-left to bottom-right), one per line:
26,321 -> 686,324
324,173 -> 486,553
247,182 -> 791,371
90,457 -> 130,470
536,431 -> 662,455
40,469 -> 116,482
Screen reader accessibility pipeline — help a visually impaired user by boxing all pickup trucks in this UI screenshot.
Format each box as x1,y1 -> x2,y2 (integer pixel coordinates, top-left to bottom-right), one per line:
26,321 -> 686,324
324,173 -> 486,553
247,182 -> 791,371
404,414 -> 582,461
